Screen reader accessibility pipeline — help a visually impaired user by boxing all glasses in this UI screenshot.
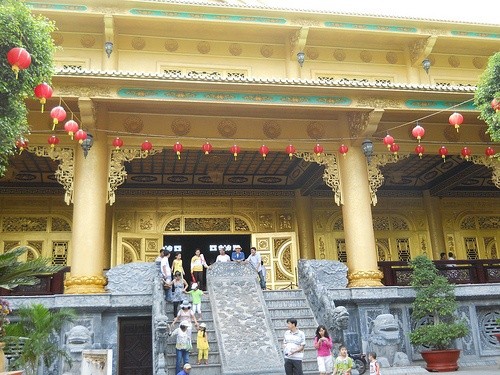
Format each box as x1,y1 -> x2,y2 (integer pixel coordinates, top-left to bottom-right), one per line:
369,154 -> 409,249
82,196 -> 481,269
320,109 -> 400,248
174,274 -> 181,277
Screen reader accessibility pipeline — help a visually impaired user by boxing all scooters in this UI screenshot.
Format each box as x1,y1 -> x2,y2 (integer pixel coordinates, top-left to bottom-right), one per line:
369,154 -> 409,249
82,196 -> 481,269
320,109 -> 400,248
349,352 -> 369,375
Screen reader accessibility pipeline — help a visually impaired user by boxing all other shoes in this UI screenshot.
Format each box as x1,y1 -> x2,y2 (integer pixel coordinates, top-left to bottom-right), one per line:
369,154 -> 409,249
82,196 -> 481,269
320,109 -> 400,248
205,362 -> 208,365
197,363 -> 201,365
198,317 -> 202,320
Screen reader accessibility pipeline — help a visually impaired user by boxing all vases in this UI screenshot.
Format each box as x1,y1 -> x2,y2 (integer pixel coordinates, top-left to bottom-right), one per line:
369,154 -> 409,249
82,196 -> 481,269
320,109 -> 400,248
0,341 -> 6,373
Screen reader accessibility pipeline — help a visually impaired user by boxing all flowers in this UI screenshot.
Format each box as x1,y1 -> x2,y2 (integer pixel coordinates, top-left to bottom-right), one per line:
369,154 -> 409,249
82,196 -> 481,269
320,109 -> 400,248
0,299 -> 13,342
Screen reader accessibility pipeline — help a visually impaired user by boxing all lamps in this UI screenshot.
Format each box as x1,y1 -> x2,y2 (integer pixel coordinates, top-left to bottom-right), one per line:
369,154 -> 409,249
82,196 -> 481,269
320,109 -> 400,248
79,133 -> 94,160
422,59 -> 431,74
297,52 -> 305,68
361,140 -> 375,167
104,42 -> 114,59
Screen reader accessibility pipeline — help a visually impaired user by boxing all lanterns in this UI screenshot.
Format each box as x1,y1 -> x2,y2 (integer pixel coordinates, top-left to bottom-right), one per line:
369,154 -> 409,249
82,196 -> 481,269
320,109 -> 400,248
383,98 -> 500,151
7,48 -> 86,154
390,143 -> 496,163
112,136 -> 349,160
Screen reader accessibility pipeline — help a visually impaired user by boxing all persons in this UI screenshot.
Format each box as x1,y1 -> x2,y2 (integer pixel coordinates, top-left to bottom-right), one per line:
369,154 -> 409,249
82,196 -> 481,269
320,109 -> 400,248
231,245 -> 245,262
172,300 -> 199,336
283,318 -> 305,375
440,252 -> 457,274
170,321 -> 193,375
332,345 -> 353,375
369,352 -> 379,375
191,249 -> 208,291
196,323 -> 209,365
245,247 -> 266,290
216,248 -> 231,262
156,249 -> 184,302
314,325 -> 334,375
164,270 -> 207,321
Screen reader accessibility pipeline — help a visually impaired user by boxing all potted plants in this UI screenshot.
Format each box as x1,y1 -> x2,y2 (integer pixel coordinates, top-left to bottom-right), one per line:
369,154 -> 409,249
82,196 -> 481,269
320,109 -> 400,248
406,255 -> 466,372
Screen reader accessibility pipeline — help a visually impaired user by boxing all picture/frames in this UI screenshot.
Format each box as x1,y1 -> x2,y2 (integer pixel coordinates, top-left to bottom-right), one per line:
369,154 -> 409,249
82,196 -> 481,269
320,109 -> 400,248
82,349 -> 112,375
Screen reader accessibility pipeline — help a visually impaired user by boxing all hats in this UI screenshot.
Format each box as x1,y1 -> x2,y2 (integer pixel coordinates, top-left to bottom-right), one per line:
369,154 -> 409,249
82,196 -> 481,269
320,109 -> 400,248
180,320 -> 191,326
199,323 -> 206,328
179,300 -> 192,308
183,363 -> 191,370
191,283 -> 198,289
234,244 -> 241,249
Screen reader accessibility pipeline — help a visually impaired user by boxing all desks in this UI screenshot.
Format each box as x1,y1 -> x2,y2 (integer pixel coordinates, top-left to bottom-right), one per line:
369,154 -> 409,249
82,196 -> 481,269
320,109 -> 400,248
0,371 -> 23,375
